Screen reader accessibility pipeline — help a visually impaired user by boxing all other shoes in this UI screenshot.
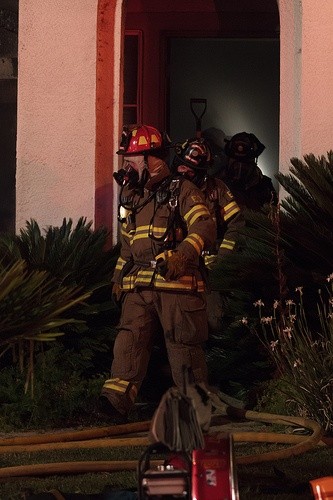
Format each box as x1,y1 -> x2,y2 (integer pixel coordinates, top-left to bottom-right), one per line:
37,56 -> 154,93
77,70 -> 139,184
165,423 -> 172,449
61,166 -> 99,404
77,394 -> 135,424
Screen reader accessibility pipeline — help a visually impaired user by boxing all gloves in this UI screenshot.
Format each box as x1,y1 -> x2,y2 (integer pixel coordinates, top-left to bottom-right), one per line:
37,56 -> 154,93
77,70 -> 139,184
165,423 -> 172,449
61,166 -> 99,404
110,281 -> 123,304
156,251 -> 189,281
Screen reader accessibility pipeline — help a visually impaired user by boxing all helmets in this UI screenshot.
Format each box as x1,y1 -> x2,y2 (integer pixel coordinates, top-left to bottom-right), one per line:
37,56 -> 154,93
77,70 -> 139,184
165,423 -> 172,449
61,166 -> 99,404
116,122 -> 164,155
174,139 -> 214,170
223,132 -> 265,159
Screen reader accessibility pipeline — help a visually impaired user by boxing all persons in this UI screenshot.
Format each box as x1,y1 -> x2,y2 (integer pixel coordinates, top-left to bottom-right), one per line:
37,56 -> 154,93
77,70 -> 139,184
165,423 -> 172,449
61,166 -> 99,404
169,131 -> 277,401
100,126 -> 215,425
